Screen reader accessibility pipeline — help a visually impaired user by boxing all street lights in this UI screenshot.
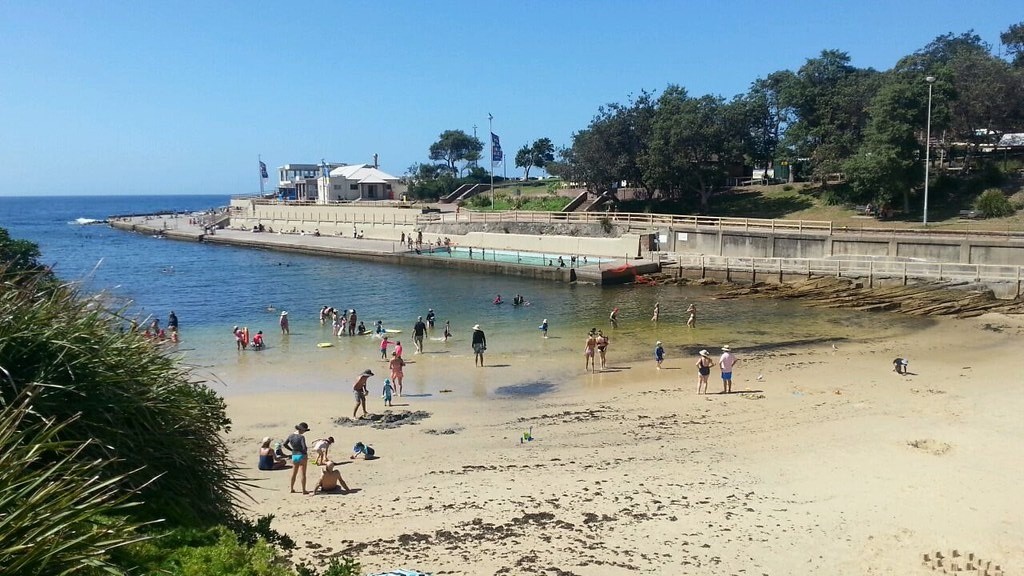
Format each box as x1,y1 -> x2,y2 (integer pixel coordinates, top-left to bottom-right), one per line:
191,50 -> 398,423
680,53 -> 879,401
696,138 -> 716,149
487,113 -> 495,209
922,73 -> 936,226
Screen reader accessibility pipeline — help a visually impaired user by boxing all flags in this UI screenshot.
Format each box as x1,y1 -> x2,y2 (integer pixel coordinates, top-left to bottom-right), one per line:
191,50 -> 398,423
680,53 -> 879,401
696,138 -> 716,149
260,162 -> 268,178
491,132 -> 503,161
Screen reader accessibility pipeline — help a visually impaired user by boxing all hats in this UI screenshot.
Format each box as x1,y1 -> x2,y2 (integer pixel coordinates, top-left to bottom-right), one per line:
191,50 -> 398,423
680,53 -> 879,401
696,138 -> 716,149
295,423 -> 310,431
473,325 -> 481,330
699,349 -> 710,356
281,310 -> 288,315
902,359 -> 908,364
543,319 -> 548,323
721,344 -> 731,351
263,436 -> 272,445
656,341 -> 662,345
362,368 -> 374,376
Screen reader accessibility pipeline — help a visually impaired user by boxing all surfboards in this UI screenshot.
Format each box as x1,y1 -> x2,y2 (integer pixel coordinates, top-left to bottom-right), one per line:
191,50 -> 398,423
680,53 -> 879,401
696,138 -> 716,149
242,327 -> 249,345
384,329 -> 403,333
317,343 -> 334,348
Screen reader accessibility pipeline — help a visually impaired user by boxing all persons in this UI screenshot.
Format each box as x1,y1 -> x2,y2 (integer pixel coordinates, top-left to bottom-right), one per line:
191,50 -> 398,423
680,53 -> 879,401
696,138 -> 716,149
610,308 -> 619,328
655,340 -> 666,369
651,303 -> 659,321
696,350 -> 714,394
144,310 -> 178,342
258,422 -> 375,496
584,328 -> 609,374
549,255 -> 587,267
494,295 -> 524,305
687,304 -> 696,328
113,209 -> 364,239
719,345 -> 737,393
233,326 -> 263,351
538,318 -> 548,337
471,324 -> 487,367
353,308 -> 449,417
280,310 -> 289,334
389,190 -> 394,199
892,358 -> 908,375
319,305 -> 382,336
400,230 -> 451,255
